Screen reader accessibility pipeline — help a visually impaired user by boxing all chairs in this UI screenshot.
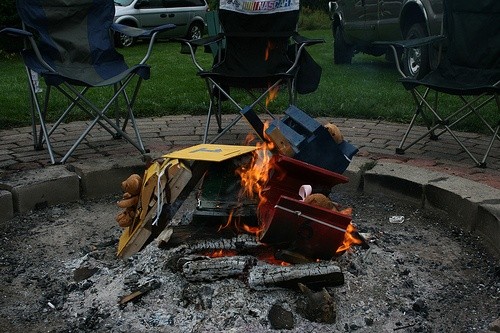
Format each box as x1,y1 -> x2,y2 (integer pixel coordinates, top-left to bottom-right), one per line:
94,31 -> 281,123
0,0 -> 176,165
169,0 -> 325,144
373,0 -> 500,169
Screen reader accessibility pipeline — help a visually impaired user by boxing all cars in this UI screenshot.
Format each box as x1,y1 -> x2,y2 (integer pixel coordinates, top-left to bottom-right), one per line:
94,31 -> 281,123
112,0 -> 211,48
327,0 -> 445,81
